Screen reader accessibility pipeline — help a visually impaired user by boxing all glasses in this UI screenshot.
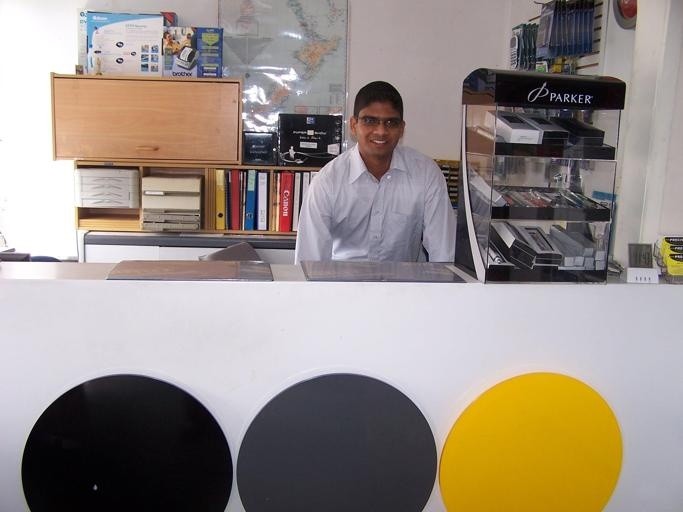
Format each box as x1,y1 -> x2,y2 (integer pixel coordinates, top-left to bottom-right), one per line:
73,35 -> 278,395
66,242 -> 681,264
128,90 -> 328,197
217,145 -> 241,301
356,116 -> 401,128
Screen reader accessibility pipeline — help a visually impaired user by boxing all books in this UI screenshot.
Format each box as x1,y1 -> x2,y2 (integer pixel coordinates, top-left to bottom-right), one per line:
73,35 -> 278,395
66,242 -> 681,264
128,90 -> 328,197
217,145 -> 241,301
216,168 -> 320,233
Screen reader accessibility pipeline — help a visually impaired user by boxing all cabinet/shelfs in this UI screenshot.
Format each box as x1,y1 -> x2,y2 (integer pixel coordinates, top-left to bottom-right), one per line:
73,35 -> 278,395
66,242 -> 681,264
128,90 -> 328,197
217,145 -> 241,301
49,71 -> 244,164
73,157 -> 482,261
454,67 -> 626,283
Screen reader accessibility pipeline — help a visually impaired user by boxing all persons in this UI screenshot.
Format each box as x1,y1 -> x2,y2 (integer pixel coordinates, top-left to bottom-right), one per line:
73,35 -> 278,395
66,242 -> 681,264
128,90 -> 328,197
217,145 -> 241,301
163,27 -> 197,54
293,81 -> 457,265
